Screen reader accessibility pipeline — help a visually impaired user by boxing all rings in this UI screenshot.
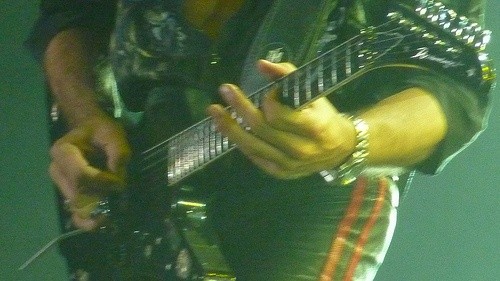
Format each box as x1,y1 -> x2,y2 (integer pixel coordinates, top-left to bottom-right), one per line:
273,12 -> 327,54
59,197 -> 75,215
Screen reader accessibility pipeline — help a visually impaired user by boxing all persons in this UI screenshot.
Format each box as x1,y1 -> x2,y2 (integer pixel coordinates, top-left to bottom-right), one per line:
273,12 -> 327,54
25,0 -> 492,281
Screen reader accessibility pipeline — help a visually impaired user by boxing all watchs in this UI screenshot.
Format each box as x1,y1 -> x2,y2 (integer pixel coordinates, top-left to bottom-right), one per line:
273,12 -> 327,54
319,110 -> 370,186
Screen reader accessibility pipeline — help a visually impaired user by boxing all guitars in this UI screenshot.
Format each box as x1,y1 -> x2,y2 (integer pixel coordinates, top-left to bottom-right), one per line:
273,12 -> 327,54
48,0 -> 495,281
